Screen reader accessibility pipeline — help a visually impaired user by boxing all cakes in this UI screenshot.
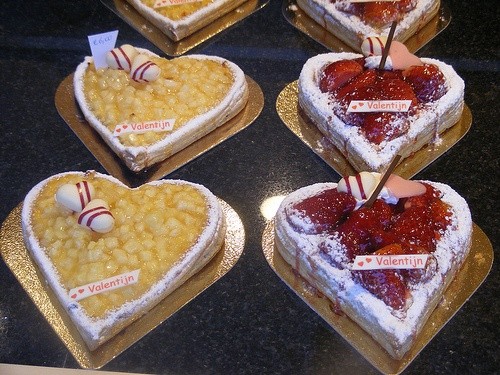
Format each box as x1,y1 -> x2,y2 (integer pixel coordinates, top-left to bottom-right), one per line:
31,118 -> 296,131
22,170 -> 227,350
73,29 -> 249,173
273,173 -> 472,360
293,0 -> 441,52
297,37 -> 465,173
127,0 -> 250,42
